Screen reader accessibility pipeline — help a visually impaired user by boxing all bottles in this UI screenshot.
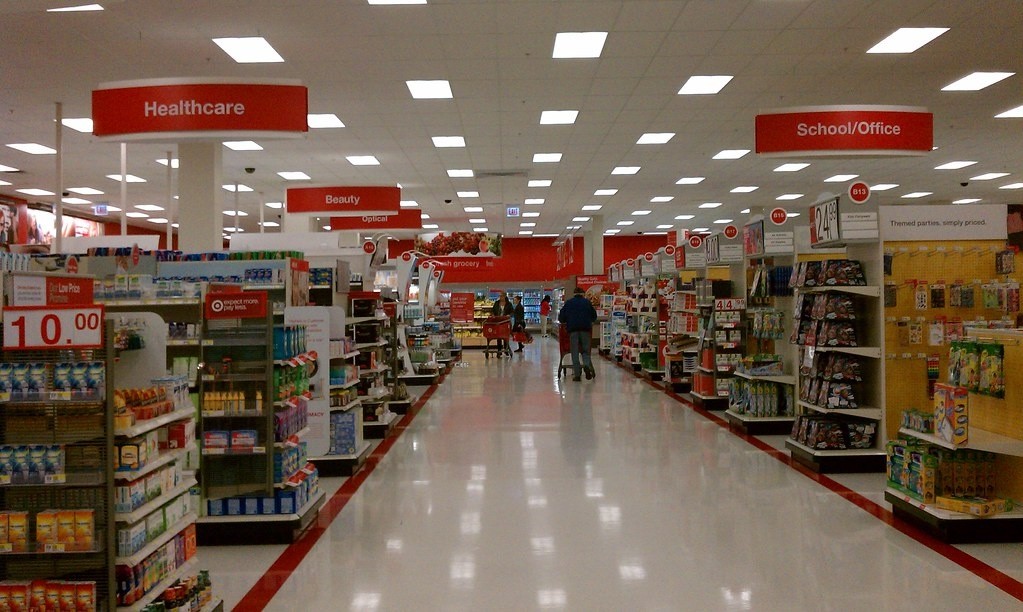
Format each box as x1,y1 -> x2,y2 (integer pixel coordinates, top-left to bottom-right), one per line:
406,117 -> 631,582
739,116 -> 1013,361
403,305 -> 424,319
139,569 -> 212,612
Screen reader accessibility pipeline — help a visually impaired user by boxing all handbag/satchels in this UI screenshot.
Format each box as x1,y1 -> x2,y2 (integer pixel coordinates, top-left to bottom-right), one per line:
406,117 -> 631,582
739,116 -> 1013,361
513,326 -> 533,344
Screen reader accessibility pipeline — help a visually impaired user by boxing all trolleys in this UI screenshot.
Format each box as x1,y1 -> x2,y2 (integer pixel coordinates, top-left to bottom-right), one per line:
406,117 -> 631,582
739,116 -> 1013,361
556,322 -> 595,379
482,315 -> 514,360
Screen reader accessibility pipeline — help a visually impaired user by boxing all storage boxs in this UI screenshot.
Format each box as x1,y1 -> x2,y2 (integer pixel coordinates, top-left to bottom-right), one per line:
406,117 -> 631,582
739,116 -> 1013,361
600,281 -> 716,396
887,343 -> 1014,517
0,247 -> 363,612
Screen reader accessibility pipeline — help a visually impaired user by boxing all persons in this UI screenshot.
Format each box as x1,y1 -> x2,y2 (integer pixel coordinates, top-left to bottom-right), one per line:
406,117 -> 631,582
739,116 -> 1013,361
540,295 -> 551,338
8,207 -> 18,244
0,210 -> 6,244
491,292 -> 515,359
558,288 -> 598,381
27,209 -> 44,245
512,296 -> 526,352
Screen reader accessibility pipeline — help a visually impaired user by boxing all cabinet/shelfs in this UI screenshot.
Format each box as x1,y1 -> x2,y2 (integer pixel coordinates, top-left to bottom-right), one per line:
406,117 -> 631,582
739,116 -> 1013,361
431,300 -> 462,357
622,284 -> 641,371
195,291 -> 326,547
597,294 -> 614,355
381,297 -> 417,414
690,270 -> 743,410
423,321 -> 457,368
725,265 -> 799,436
284,305 -> 373,476
882,328 -> 1023,541
348,290 -> 399,439
785,260 -> 885,474
404,303 -> 447,374
641,279 -> 668,381
609,291 -> 627,362
663,267 -> 697,391
0,311 -> 224,612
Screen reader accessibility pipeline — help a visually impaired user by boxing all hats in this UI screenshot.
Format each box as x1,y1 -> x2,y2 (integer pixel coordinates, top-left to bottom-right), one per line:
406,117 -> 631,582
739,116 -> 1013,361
499,292 -> 507,296
574,287 -> 585,293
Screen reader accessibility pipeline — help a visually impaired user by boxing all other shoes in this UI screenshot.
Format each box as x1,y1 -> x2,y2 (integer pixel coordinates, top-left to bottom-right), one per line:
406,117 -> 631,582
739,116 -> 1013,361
572,377 -> 581,381
519,345 -> 524,350
497,352 -> 502,356
583,365 -> 592,380
504,352 -> 508,356
514,349 -> 522,352
542,334 -> 550,338
